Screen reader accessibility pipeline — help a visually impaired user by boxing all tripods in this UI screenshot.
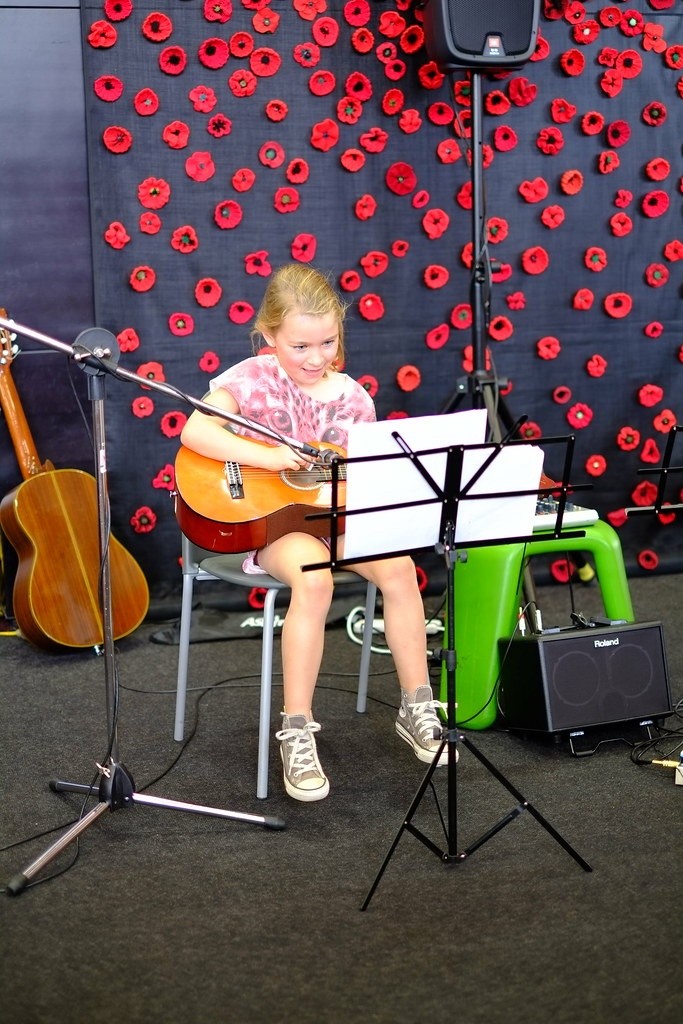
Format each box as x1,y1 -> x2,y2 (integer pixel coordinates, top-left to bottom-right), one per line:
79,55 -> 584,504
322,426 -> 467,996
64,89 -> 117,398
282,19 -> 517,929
301,432 -> 596,914
442,70 -> 596,636
0,317 -> 327,898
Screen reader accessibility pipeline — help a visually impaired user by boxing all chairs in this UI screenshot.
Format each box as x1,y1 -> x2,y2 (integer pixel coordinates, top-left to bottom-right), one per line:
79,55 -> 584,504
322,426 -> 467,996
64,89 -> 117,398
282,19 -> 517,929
173,531 -> 377,798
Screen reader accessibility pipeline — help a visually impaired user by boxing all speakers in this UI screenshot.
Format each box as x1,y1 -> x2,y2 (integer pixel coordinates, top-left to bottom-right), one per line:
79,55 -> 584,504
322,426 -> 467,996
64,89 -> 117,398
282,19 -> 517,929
423,0 -> 541,72
497,620 -> 674,759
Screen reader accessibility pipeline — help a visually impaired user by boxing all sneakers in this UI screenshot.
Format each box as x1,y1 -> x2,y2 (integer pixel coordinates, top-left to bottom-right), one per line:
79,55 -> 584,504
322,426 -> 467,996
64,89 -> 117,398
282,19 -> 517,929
275,705 -> 329,802
394,685 -> 459,765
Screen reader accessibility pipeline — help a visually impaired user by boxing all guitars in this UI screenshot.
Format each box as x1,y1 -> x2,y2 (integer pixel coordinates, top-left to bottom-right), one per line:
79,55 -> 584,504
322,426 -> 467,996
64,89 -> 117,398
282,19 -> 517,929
0,307 -> 150,654
171,434 -> 557,556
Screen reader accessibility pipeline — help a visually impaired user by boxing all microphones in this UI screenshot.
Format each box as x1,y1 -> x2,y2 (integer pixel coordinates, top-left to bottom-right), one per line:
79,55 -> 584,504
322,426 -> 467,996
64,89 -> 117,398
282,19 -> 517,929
317,447 -> 345,469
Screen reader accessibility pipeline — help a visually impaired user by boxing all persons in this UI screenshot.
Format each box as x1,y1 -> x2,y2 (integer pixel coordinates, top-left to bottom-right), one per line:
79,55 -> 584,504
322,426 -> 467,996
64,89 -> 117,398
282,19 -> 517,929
181,263 -> 462,802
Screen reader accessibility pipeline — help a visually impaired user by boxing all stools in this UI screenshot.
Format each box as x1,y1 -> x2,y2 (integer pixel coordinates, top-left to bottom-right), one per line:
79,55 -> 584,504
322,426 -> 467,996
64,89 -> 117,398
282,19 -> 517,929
439,520 -> 635,730
